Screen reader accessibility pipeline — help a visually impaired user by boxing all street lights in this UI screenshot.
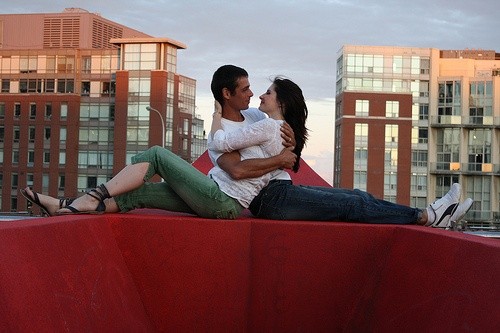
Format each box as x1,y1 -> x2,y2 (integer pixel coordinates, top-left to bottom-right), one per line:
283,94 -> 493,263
146,106 -> 166,181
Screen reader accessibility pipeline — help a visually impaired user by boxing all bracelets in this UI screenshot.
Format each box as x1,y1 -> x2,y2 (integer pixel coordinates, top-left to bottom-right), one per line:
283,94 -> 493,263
212,111 -> 222,117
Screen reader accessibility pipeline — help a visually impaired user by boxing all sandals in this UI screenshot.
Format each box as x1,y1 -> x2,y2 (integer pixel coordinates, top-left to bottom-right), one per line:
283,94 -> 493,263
57,184 -> 111,213
20,186 -> 75,215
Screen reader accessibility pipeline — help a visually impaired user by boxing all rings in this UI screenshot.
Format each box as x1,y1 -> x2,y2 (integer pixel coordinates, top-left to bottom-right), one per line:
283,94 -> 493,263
289,137 -> 292,143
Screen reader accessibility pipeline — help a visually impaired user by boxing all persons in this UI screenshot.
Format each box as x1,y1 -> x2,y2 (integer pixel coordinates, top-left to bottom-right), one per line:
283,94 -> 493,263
207,64 -> 474,231
18,74 -> 308,219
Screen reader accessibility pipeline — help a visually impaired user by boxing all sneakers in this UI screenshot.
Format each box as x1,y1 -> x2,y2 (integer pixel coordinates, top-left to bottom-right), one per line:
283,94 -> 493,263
447,197 -> 474,229
429,183 -> 460,227
